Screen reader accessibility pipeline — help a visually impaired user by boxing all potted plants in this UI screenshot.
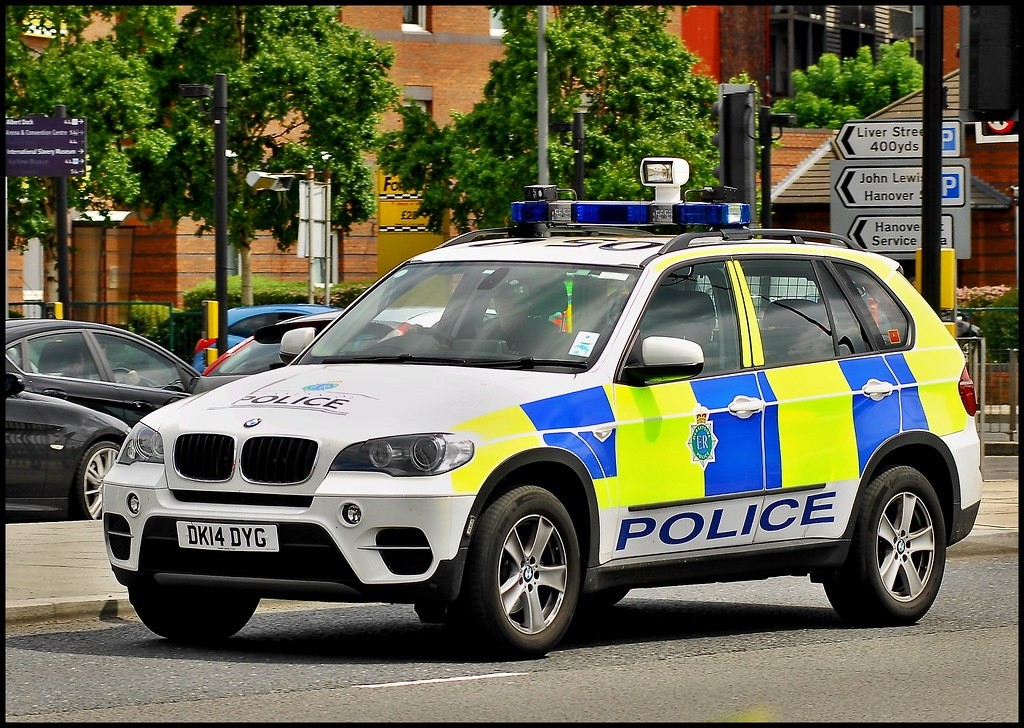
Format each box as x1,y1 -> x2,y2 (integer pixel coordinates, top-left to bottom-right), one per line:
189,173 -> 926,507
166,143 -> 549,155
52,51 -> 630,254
979,288 -> 1018,402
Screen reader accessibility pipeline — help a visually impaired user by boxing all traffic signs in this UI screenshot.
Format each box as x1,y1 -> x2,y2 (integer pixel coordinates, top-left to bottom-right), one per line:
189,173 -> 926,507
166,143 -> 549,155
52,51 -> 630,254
845,213 -> 954,253
833,117 -> 966,157
834,160 -> 966,210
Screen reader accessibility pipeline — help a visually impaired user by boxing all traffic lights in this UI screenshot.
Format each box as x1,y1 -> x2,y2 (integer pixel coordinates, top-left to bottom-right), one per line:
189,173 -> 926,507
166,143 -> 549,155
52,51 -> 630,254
709,83 -> 756,223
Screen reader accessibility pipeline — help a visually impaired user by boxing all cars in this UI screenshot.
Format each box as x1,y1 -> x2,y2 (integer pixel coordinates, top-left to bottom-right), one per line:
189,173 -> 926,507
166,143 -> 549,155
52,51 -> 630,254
2,315 -> 202,435
97,156 -> 989,655
189,303 -> 339,376
2,369 -> 137,526
183,303 -> 499,407
941,311 -> 985,366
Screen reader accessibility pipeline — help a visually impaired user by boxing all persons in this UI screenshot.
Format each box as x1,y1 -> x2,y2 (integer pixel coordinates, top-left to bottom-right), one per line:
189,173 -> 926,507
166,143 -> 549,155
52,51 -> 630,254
405,279 -> 553,355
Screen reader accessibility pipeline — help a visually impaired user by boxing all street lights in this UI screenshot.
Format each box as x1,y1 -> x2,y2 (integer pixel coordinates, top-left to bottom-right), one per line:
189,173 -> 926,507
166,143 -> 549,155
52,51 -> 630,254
175,70 -> 230,356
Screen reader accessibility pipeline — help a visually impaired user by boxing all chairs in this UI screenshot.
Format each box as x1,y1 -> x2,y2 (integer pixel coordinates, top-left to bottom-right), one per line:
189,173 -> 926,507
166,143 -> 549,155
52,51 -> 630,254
528,281 -> 572,347
763,299 -> 820,357
675,291 -> 715,353
